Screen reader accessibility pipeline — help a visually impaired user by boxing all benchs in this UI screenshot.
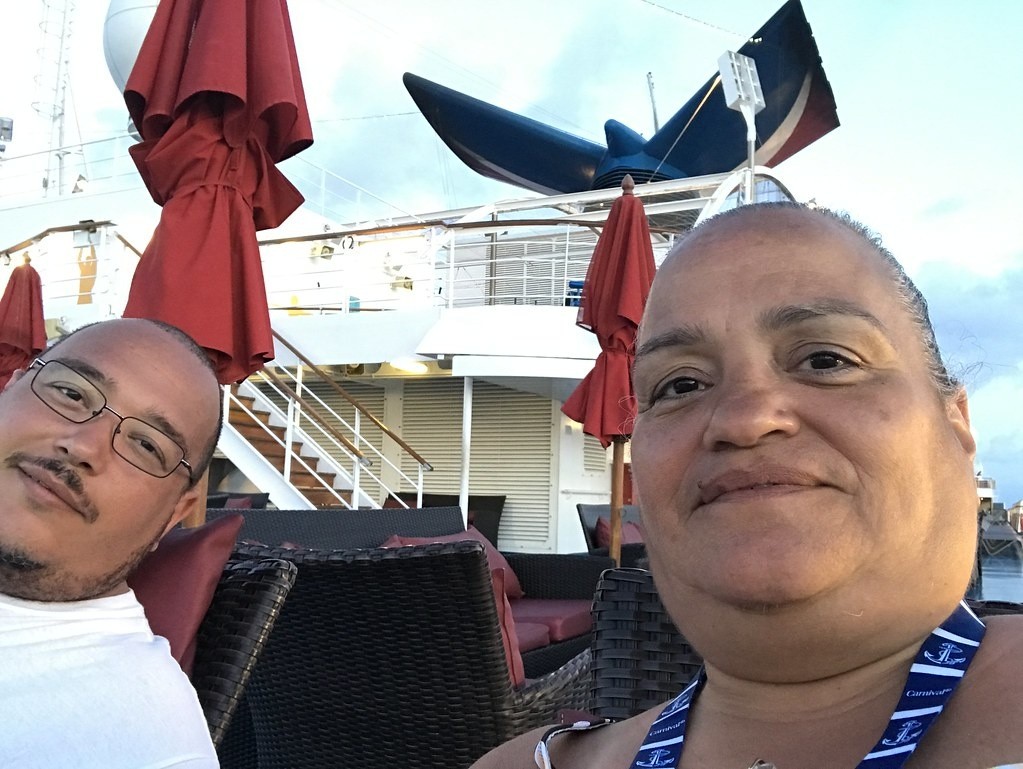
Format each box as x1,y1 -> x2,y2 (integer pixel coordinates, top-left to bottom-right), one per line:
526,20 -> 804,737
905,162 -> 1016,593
379,491 -> 507,553
575,502 -> 646,552
206,492 -> 269,510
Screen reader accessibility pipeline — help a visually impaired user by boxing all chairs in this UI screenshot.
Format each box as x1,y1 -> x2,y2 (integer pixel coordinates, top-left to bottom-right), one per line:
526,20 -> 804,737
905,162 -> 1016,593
174,505 -> 617,684
228,536 -> 597,769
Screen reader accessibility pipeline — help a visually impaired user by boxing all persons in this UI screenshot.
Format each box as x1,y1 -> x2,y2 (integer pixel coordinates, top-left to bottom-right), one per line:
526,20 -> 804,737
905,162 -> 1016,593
462,196 -> 1023,767
0,314 -> 229,769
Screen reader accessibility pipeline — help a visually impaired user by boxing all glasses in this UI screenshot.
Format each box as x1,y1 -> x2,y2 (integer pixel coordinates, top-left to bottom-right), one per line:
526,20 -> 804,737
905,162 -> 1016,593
26,358 -> 195,491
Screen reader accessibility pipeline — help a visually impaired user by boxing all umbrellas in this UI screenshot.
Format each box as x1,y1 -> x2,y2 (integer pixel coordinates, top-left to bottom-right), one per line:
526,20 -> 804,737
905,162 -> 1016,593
0,251 -> 48,391
558,171 -> 660,570
119,1 -> 318,531
